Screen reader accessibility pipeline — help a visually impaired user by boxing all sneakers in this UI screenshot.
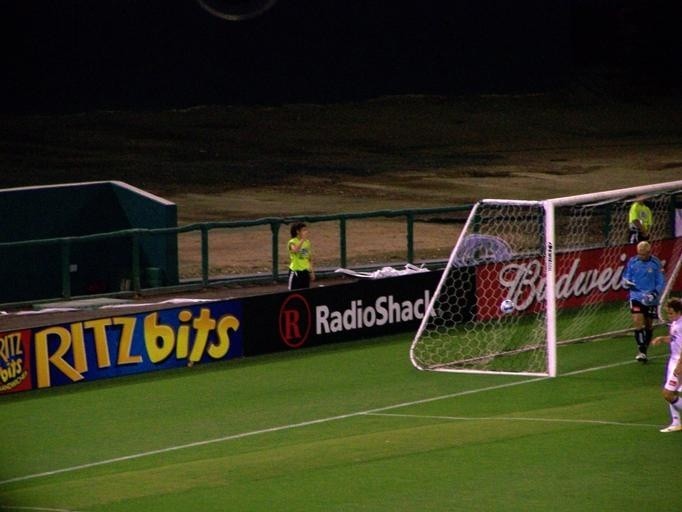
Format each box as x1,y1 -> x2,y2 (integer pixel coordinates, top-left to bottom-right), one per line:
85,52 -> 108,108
659,424 -> 682,433
636,352 -> 647,360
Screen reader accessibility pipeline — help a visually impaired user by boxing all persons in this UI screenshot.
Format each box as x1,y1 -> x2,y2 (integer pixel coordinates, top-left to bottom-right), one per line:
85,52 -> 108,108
621,241 -> 664,362
287,222 -> 316,290
651,297 -> 682,432
628,195 -> 653,243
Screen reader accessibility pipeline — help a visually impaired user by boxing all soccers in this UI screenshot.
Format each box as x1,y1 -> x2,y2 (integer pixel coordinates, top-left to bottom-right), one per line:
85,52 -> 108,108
500,300 -> 514,314
